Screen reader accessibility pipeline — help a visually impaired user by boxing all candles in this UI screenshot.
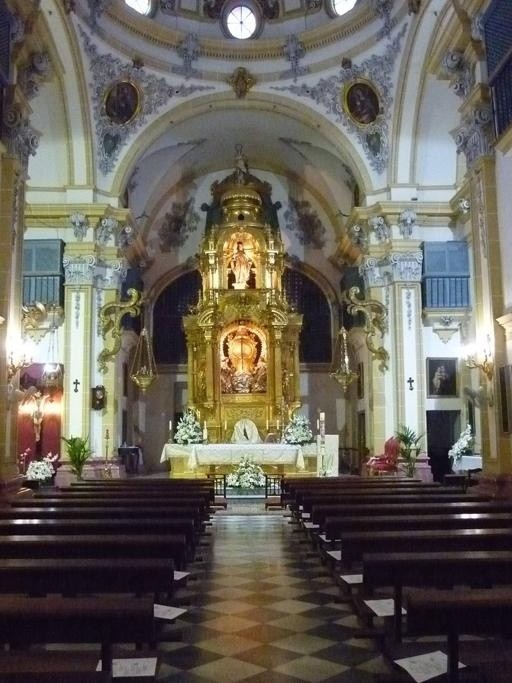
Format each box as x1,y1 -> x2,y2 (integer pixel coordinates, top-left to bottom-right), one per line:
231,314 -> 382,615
168,419 -> 172,431
316,419 -> 320,430
203,420 -> 206,429
265,419 -> 280,430
224,420 -> 227,431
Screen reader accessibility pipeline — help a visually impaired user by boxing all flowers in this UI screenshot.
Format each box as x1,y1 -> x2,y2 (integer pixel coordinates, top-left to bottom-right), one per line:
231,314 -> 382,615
172,409 -> 203,444
226,453 -> 280,490
282,414 -> 313,443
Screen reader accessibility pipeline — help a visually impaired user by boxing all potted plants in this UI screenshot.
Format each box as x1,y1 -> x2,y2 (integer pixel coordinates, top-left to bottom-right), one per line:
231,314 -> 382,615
395,423 -> 425,478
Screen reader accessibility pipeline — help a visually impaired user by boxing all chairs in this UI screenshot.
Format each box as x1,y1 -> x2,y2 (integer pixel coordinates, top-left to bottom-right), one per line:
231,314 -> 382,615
365,434 -> 402,477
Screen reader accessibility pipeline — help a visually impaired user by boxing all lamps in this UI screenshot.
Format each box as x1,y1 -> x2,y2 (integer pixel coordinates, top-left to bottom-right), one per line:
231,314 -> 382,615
127,327 -> 160,396
327,311 -> 361,393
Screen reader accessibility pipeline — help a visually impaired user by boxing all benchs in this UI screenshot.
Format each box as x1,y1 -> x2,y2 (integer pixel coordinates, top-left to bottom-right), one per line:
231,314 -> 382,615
1,476 -> 228,683
264,475 -> 512,683
